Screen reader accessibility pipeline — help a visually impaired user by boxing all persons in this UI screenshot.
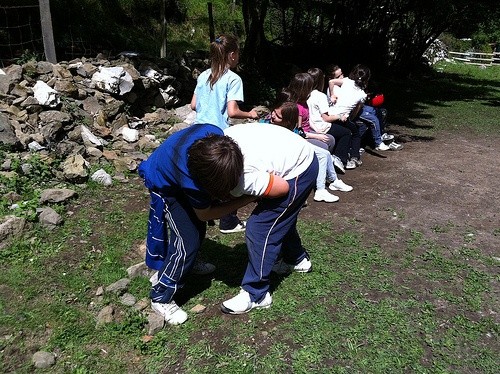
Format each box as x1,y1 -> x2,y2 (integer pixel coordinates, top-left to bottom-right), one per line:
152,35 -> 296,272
358,105 -> 390,151
324,64 -> 368,154
306,68 -> 352,174
327,64 -> 372,170
136,124 -> 245,327
190,33 -> 261,232
220,122 -> 319,315
367,98 -> 404,151
253,102 -> 299,138
284,72 -> 353,203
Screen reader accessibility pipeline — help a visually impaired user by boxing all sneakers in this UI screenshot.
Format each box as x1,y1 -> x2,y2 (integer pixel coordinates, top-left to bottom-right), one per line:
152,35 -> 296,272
314,188 -> 339,202
219,290 -> 274,314
345,160 -> 357,169
380,133 -> 394,142
350,156 -> 362,167
272,258 -> 312,272
151,297 -> 188,326
329,154 -> 345,174
375,142 -> 389,150
329,179 -> 354,191
390,142 -> 403,150
220,221 -> 247,234
